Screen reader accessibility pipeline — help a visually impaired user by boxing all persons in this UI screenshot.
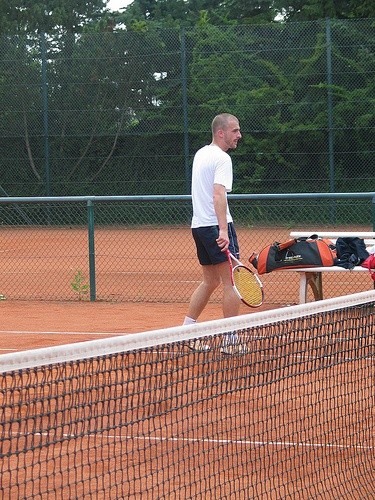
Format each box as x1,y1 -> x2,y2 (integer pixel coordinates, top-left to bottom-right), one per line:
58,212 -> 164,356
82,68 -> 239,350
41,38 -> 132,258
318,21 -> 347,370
178,113 -> 251,355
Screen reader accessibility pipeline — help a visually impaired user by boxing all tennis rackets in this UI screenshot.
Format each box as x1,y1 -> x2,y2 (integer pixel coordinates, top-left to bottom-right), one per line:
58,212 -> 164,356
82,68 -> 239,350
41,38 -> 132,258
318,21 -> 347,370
222,246 -> 265,309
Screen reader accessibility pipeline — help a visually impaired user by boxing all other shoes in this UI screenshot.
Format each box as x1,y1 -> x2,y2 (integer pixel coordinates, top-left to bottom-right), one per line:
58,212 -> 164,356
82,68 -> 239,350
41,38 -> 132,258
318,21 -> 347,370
180,339 -> 210,352
220,338 -> 250,354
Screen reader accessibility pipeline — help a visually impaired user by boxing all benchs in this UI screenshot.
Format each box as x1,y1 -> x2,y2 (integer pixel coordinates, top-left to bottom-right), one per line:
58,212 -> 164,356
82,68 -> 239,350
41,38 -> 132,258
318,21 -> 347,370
273,231 -> 375,306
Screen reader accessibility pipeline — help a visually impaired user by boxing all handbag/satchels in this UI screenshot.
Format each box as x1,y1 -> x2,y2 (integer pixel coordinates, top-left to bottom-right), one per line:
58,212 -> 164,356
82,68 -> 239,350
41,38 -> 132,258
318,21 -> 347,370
361,253 -> 375,280
248,234 -> 337,275
